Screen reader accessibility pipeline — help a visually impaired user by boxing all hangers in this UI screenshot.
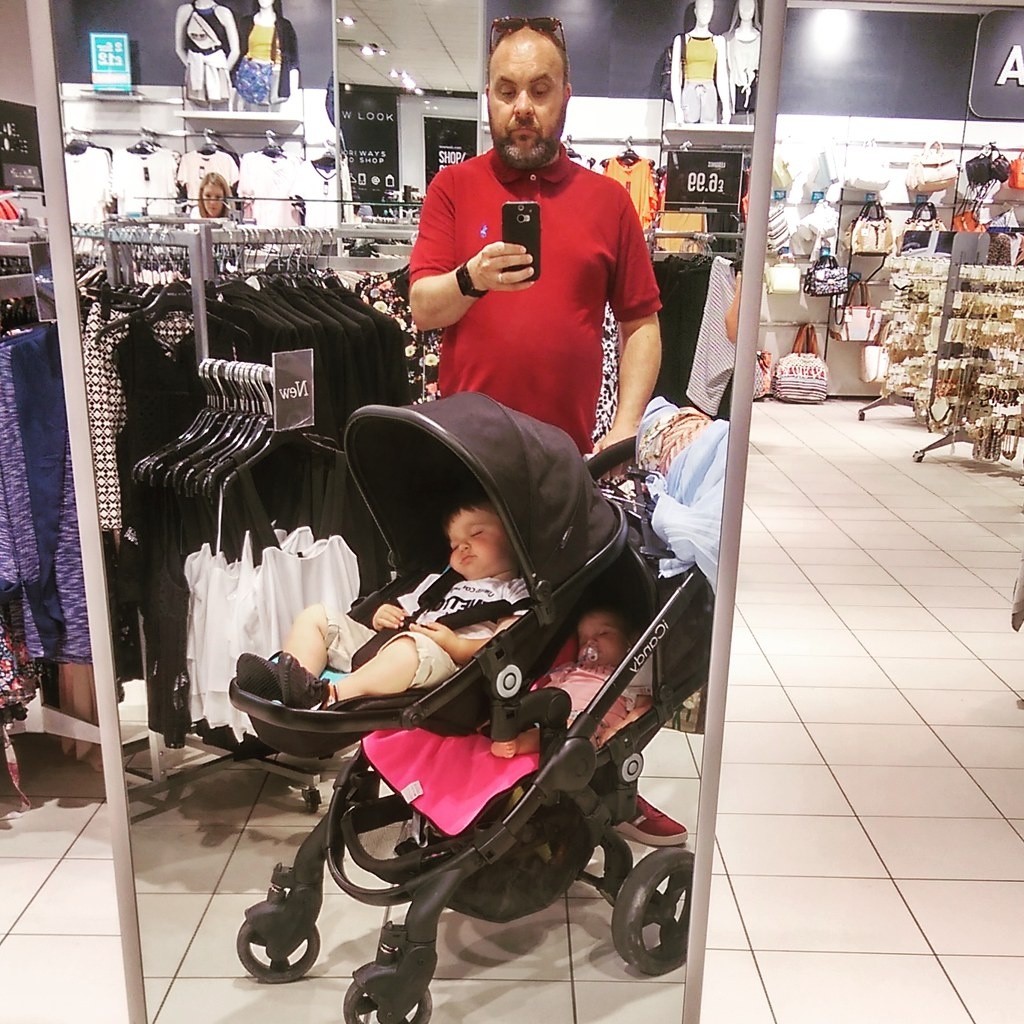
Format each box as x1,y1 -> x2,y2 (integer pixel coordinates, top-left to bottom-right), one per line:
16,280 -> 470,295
64,216 -> 410,339
58,126 -> 345,175
132,356 -> 345,501
561,133 -> 656,172
664,234 -> 732,274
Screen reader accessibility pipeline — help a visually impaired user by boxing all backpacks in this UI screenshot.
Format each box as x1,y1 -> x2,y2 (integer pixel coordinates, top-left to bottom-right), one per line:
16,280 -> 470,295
660,34 -> 686,102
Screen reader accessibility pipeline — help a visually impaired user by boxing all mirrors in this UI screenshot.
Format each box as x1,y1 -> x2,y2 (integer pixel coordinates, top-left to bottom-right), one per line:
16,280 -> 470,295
30,0 -> 786,1024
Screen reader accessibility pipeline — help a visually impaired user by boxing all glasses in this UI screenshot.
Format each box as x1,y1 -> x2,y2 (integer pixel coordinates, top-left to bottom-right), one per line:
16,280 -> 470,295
488,15 -> 566,55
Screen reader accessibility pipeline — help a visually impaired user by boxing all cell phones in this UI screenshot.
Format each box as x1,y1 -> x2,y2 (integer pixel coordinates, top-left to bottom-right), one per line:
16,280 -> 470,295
502,202 -> 541,281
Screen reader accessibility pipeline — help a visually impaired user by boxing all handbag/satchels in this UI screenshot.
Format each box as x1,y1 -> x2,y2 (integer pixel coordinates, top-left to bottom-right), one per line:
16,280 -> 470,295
906,140 -> 957,192
829,281 -> 884,342
233,56 -> 273,107
753,323 -> 828,403
954,148 -> 1024,230
768,139 -> 946,257
764,256 -> 801,295
805,255 -> 848,295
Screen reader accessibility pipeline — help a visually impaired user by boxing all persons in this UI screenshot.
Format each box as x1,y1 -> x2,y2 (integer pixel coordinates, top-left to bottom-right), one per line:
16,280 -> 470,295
232,0 -> 300,105
236,492 -> 529,710
720,0 -> 762,116
175,0 -> 240,103
198,173 -> 231,218
671,0 -> 730,126
490,602 -> 632,760
409,17 -> 688,846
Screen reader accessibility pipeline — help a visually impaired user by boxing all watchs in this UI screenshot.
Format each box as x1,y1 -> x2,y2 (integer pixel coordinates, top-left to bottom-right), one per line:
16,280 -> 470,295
456,262 -> 489,298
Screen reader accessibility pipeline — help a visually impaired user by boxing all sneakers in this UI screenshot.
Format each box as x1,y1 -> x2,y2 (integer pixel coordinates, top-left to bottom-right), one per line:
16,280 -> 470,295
614,797 -> 688,846
237,653 -> 282,703
278,653 -> 329,711
